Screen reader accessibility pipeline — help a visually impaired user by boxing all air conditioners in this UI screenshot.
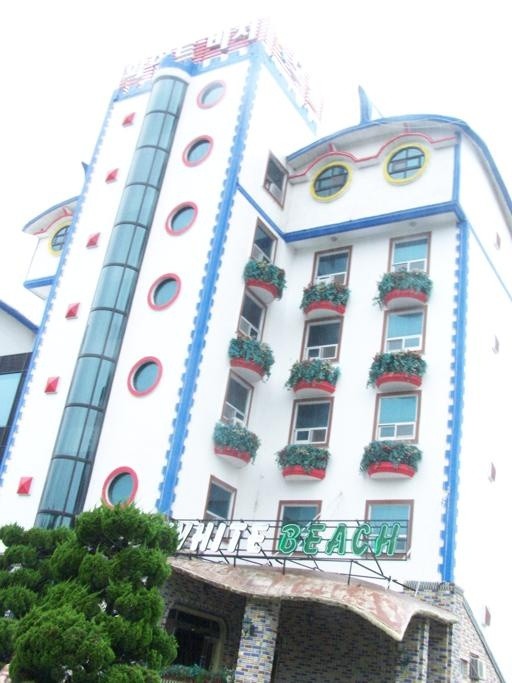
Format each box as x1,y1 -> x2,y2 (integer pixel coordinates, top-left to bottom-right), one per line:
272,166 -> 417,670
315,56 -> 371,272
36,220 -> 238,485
471,657 -> 486,681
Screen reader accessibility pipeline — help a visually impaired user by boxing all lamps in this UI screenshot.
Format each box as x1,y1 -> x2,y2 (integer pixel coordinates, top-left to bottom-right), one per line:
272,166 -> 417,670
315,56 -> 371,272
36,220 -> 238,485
241,615 -> 255,639
